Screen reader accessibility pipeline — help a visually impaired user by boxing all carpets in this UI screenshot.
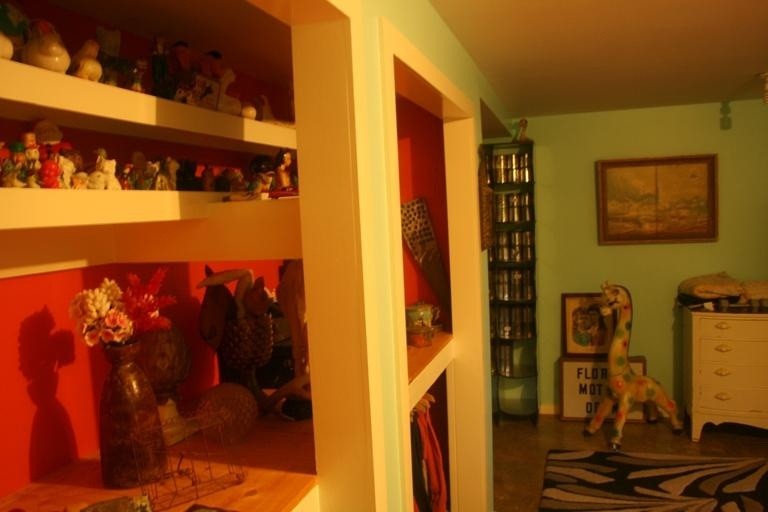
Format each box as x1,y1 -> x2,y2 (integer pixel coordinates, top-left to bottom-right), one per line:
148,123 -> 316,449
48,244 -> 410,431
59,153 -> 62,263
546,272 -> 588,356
538,449 -> 768,512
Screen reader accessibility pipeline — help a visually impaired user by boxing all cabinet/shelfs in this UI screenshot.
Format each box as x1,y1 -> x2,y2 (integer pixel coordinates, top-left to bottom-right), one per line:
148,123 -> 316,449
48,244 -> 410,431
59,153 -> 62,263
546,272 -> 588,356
692,312 -> 768,417
483,153 -> 535,379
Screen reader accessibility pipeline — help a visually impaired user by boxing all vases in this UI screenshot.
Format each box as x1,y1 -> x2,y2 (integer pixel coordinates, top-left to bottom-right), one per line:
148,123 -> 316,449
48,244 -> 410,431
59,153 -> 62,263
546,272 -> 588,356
98,346 -> 168,489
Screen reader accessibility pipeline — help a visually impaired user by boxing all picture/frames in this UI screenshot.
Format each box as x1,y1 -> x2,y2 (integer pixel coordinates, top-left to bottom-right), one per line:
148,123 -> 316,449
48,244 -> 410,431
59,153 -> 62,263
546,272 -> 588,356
559,356 -> 648,424
595,153 -> 717,245
562,291 -> 617,357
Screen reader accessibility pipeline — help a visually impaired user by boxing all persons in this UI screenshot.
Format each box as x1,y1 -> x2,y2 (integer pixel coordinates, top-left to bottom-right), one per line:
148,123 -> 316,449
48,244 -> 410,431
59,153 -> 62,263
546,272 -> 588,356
24,133 -> 42,189
8,143 -> 26,183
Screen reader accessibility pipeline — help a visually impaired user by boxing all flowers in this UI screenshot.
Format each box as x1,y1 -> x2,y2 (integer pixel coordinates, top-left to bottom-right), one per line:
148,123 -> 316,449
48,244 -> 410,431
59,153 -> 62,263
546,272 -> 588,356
73,273 -> 176,345
